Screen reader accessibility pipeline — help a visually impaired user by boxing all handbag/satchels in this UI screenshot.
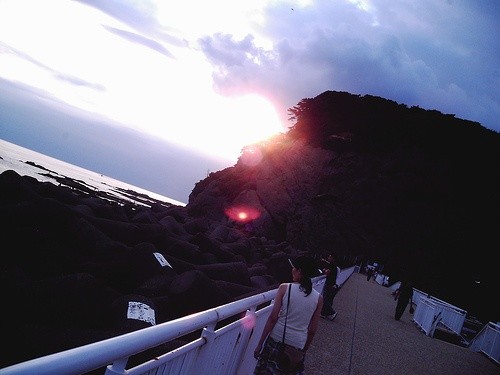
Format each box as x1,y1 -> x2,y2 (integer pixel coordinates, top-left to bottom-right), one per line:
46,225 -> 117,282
278,343 -> 305,373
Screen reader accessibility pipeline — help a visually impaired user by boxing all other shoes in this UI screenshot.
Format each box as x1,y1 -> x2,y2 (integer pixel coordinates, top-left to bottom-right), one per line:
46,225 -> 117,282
330,312 -> 338,320
320,314 -> 329,319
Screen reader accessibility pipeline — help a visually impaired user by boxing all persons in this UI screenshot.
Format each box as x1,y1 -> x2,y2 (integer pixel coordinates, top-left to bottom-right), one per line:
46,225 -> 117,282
367,267 -> 374,281
253,256 -> 323,375
320,253 -> 338,321
395,279 -> 413,320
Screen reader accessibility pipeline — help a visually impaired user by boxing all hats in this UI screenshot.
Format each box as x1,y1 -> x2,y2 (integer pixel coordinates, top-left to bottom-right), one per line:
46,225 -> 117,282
288,256 -> 314,272
